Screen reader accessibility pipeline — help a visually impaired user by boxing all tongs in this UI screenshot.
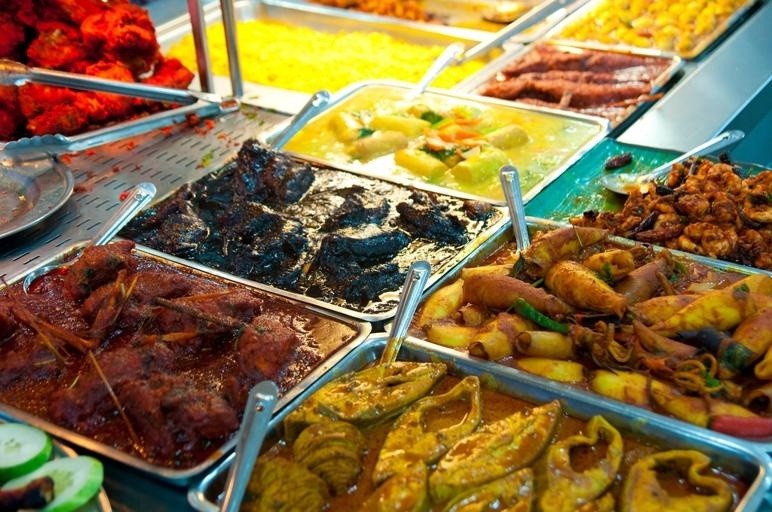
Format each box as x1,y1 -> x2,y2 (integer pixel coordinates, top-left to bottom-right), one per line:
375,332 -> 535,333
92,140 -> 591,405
0,55 -> 224,166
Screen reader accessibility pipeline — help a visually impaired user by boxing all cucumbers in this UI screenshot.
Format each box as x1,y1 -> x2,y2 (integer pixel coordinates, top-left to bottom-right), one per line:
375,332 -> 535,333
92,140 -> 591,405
0,423 -> 104,512
